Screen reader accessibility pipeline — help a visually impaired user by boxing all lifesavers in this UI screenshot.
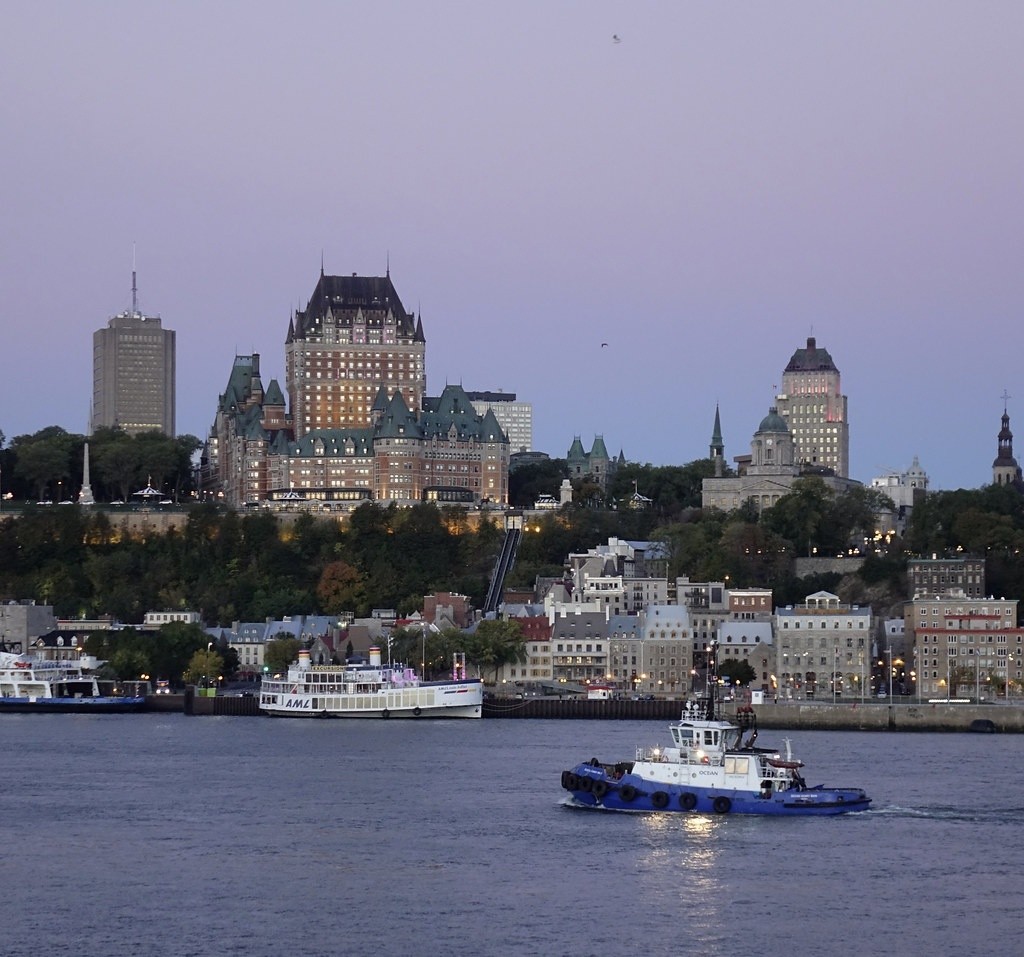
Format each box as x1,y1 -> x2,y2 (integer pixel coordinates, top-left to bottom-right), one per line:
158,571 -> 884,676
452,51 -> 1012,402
591,780 -> 608,797
382,709 -> 391,718
679,792 -> 697,810
561,770 -> 570,789
567,774 -> 579,791
579,776 -> 593,792
713,796 -> 731,814
618,786 -> 636,802
652,790 -> 668,808
413,708 -> 421,715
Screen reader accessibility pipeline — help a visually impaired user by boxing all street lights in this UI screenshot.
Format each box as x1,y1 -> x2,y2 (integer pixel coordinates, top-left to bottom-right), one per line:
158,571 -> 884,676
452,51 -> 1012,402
207,641 -> 213,688
770,675 -> 779,704
706,640 -> 720,693
218,675 -> 222,693
634,674 -> 647,692
690,670 -> 697,692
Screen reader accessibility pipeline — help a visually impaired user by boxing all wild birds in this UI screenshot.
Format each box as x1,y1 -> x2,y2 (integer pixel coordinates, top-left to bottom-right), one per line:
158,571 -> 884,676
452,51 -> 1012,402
601,344 -> 607,347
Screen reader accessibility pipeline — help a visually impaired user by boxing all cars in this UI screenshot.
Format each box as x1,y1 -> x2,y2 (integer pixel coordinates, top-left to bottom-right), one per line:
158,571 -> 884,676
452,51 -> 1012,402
36,499 -> 173,505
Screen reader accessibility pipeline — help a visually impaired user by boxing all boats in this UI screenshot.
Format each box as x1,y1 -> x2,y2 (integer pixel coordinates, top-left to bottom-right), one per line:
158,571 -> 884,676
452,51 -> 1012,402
561,692 -> 872,816
258,645 -> 483,719
0,645 -> 152,713
